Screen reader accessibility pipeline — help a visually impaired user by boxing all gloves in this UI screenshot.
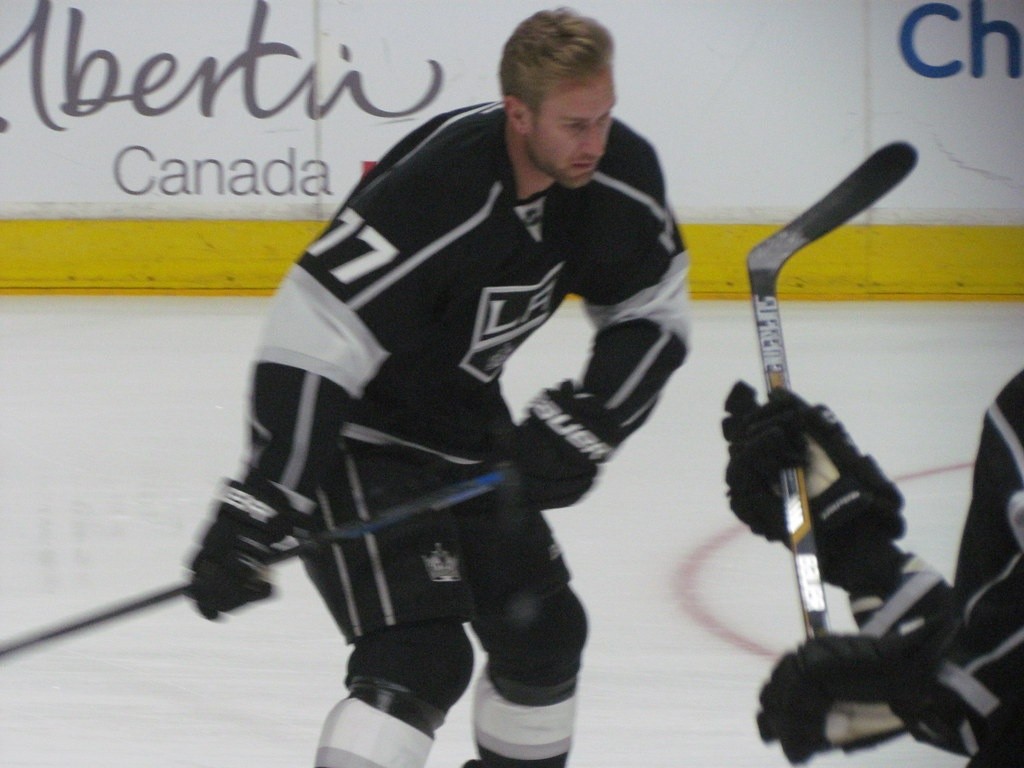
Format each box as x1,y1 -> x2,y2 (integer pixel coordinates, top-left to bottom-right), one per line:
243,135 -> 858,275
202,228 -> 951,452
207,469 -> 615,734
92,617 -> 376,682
191,475 -> 314,620
513,381 -> 618,511
720,380 -> 906,590
757,631 -> 943,765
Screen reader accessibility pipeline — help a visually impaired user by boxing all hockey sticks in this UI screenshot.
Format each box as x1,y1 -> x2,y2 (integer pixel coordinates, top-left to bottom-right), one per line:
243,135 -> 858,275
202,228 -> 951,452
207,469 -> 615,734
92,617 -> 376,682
0,463 -> 518,660
747,141 -> 919,644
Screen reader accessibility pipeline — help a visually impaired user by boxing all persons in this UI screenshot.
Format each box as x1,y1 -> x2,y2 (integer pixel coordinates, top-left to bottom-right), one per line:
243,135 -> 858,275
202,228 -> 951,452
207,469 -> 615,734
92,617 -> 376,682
190,9 -> 690,768
722,373 -> 1024,768
